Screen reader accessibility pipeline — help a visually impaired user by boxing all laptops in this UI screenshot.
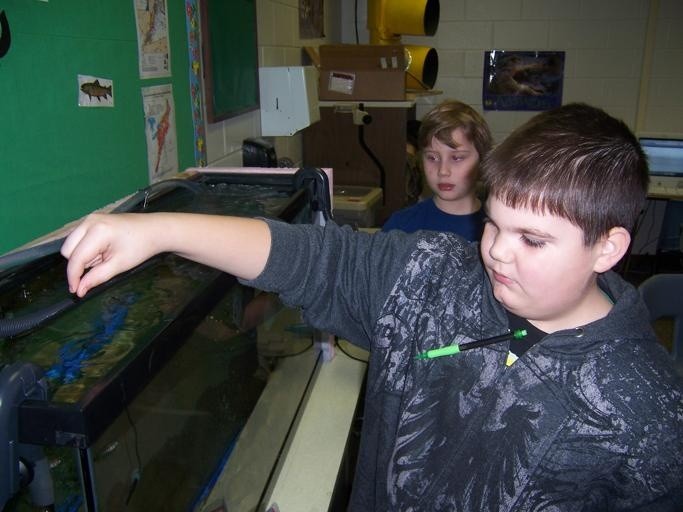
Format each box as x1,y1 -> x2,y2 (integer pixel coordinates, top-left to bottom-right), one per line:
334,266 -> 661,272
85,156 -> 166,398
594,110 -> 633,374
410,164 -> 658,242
636,132 -> 683,197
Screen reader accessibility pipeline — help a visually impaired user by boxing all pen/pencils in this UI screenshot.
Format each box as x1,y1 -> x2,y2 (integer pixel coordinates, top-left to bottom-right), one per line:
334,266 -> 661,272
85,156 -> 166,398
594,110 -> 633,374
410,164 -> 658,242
412,329 -> 527,360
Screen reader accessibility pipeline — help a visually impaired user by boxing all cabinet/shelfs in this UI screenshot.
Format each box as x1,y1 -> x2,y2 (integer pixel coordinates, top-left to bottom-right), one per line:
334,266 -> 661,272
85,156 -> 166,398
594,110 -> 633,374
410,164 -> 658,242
313,104 -> 417,226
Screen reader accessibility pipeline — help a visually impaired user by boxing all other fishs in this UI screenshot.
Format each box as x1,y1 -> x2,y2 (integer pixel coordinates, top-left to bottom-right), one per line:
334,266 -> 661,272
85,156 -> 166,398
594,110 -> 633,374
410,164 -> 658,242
101,440 -> 118,455
81,80 -> 113,102
49,459 -> 62,470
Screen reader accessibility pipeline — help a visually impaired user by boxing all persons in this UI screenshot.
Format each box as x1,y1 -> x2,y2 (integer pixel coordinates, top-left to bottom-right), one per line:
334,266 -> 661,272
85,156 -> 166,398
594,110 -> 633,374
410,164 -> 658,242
57,100 -> 682,511
379,100 -> 494,242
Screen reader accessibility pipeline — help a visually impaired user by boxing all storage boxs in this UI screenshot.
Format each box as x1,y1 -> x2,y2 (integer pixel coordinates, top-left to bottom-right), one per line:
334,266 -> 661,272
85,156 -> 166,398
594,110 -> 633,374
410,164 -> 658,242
317,44 -> 406,100
